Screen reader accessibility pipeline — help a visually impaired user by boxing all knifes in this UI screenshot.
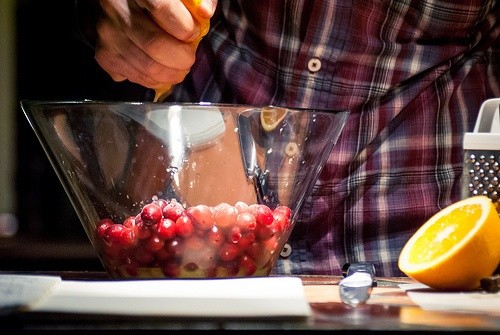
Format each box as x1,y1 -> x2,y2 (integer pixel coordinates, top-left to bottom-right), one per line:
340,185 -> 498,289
338,263 -> 376,309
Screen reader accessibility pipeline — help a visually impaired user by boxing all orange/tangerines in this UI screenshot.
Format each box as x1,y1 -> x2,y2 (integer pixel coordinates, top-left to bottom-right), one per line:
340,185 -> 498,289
398,195 -> 500,291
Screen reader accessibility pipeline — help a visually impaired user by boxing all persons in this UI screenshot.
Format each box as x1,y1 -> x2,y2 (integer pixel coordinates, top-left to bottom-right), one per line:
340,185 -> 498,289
95,0 -> 500,275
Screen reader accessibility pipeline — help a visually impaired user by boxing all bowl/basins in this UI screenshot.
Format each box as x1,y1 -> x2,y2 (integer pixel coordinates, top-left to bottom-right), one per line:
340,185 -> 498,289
19,99 -> 352,280
